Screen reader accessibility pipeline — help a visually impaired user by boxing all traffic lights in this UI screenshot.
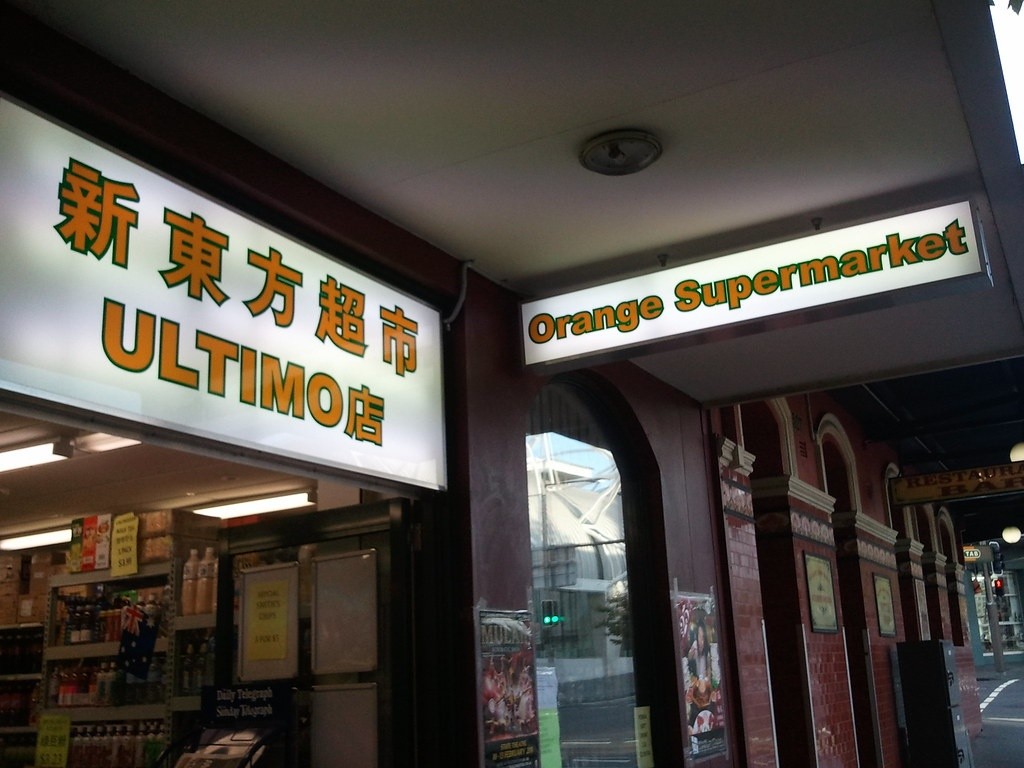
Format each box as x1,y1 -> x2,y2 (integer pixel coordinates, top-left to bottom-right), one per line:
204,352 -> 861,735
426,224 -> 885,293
542,600 -> 553,628
995,580 -> 1004,595
553,601 -> 558,626
989,542 -> 1005,575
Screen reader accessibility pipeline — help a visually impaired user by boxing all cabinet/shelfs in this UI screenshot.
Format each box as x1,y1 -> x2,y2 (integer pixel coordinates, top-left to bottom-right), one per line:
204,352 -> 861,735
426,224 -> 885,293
172,608 -> 219,713
37,556 -> 181,766
0,620 -> 44,733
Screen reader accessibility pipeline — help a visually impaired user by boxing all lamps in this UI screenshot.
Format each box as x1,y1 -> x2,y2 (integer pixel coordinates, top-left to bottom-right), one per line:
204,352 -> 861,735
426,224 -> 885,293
0,442 -> 74,473
1003,526 -> 1021,544
192,488 -> 316,520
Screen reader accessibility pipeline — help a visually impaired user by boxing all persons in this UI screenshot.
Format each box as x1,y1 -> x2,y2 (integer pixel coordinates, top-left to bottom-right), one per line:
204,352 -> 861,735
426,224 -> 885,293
688,622 -> 711,727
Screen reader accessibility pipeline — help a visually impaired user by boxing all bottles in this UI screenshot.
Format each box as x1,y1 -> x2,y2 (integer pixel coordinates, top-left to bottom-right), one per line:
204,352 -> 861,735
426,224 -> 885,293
181,639 -> 216,691
181,549 -> 198,615
194,548 -> 214,614
36,590 -> 176,768
209,546 -> 218,612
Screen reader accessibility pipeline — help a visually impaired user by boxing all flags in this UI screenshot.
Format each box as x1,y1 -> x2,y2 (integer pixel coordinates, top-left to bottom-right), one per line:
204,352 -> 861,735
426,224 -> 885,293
116,600 -> 158,680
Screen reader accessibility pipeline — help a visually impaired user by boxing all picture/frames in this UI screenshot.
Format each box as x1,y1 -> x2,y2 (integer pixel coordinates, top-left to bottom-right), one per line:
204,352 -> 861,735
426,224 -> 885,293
802,550 -> 839,634
871,571 -> 897,637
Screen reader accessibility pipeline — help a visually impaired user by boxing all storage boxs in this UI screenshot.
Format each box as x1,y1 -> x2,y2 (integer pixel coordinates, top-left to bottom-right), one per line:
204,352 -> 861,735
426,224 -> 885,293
0,510 -> 223,624
